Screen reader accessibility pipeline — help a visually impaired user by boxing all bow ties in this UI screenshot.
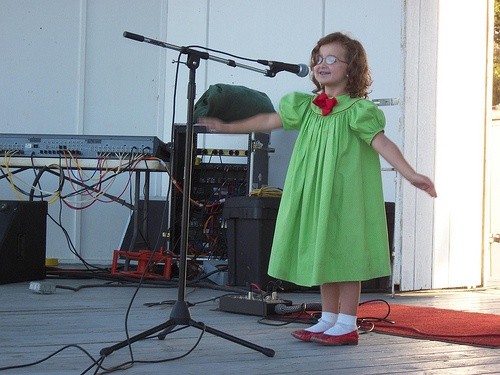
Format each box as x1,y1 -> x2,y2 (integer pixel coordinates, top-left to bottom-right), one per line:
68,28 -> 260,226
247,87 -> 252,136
312,92 -> 338,116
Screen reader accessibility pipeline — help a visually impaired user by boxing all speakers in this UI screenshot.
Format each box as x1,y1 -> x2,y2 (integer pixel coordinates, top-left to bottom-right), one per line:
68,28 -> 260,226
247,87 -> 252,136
0,200 -> 48,284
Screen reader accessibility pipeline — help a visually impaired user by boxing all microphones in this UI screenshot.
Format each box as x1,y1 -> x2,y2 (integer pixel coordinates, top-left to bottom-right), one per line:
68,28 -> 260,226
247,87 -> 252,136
257,59 -> 309,77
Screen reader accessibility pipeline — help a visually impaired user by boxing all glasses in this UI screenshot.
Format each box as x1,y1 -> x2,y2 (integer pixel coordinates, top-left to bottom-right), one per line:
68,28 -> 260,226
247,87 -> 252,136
315,54 -> 349,64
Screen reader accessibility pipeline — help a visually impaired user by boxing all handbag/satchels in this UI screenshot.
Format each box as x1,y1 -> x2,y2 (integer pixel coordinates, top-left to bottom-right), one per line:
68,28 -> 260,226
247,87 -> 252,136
192,83 -> 277,127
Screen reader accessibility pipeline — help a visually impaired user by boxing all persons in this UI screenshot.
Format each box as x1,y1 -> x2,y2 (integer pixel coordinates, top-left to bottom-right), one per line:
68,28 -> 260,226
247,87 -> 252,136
198,32 -> 437,345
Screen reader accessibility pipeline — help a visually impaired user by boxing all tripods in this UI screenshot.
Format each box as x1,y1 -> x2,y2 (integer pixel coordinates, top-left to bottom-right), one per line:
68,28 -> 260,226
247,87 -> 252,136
99,30 -> 275,357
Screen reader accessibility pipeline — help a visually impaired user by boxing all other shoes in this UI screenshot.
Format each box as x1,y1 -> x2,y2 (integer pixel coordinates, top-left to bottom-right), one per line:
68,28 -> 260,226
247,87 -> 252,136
291,329 -> 323,341
310,329 -> 358,345
